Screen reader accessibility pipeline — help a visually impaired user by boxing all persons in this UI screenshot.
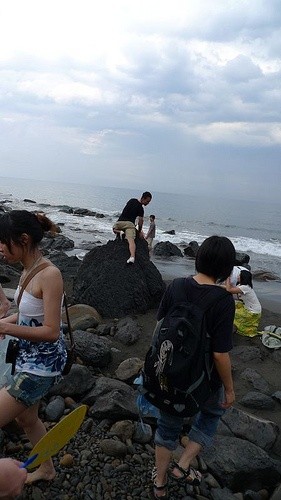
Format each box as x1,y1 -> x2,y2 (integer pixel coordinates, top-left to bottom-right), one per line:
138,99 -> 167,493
142,236 -> 236,500
113,191 -> 152,264
225,270 -> 262,338
144,215 -> 155,247
230,260 -> 249,288
0,209 -> 68,500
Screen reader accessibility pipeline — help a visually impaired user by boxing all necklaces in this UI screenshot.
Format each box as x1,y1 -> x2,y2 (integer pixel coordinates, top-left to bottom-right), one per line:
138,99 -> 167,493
15,253 -> 42,292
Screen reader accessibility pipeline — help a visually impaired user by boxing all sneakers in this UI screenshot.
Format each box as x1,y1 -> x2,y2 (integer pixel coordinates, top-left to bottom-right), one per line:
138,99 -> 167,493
120,231 -> 125,240
127,257 -> 134,263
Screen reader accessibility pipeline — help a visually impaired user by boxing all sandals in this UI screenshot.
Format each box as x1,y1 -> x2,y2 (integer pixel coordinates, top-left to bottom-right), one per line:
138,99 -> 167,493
151,467 -> 169,500
169,462 -> 202,485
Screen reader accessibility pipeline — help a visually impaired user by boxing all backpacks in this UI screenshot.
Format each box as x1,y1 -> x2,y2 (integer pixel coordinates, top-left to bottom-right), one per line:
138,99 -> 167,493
143,278 -> 226,403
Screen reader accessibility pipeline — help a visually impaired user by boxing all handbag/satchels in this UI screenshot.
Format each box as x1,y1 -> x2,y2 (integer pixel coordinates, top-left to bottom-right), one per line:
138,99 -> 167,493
262,325 -> 281,349
5,339 -> 73,376
